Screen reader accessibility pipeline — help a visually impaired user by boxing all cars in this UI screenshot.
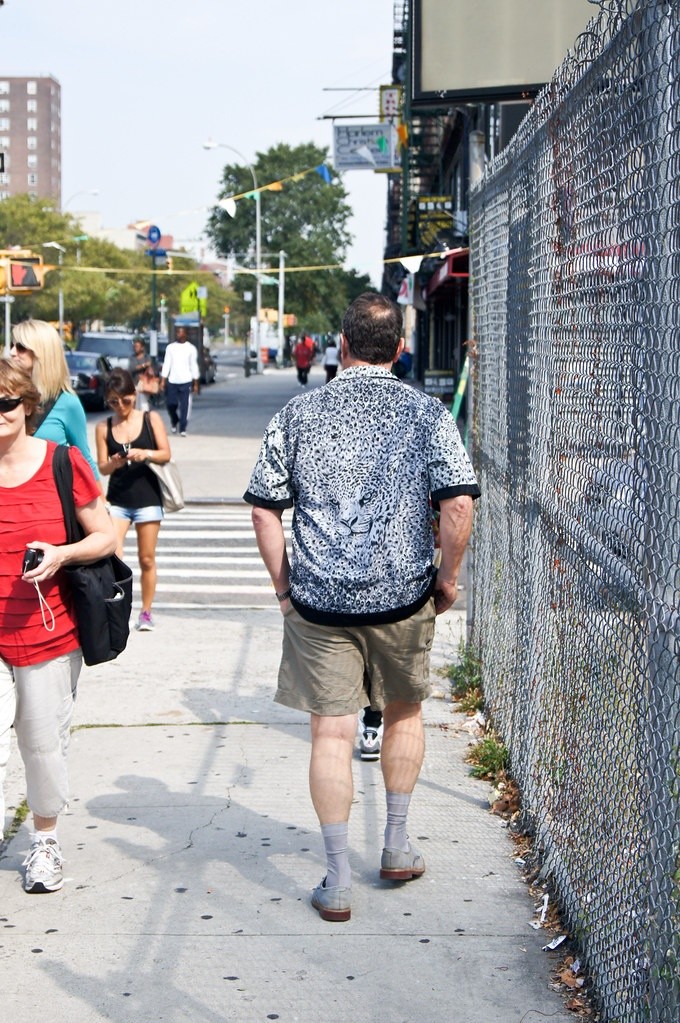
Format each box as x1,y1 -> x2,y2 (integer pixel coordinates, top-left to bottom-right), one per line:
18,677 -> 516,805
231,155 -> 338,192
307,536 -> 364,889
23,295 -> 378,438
67,351 -> 112,411
144,336 -> 168,376
197,345 -> 218,384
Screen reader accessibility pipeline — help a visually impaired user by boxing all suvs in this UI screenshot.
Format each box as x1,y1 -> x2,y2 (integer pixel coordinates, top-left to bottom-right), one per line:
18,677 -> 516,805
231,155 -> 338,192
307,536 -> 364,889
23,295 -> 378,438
80,333 -> 148,385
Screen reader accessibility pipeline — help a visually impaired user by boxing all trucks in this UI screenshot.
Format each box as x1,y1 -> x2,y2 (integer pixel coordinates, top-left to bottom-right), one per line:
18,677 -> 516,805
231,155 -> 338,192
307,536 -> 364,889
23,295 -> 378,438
249,316 -> 285,357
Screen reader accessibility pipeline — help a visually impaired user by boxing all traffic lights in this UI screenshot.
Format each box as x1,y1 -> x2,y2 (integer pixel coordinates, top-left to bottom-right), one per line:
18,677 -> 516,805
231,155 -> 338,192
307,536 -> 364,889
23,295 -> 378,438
0,249 -> 42,296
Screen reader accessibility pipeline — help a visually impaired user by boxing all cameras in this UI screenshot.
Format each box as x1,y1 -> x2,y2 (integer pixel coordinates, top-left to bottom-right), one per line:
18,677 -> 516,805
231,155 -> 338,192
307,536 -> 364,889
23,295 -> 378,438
22,548 -> 43,574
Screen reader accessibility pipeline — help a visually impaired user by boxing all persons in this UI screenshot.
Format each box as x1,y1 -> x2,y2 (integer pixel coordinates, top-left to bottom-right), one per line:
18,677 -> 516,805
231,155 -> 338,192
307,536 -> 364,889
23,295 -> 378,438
0,318 -> 218,892
243,294 -> 485,922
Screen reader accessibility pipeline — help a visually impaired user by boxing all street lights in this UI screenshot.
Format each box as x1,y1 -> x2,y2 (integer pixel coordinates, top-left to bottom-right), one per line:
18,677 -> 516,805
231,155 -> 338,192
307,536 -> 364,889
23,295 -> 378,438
203,143 -> 263,375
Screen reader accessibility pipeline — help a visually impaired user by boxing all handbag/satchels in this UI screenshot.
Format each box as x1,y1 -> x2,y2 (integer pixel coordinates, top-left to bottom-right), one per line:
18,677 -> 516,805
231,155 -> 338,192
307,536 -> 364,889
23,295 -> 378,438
138,367 -> 159,394
52,444 -> 134,666
147,460 -> 185,513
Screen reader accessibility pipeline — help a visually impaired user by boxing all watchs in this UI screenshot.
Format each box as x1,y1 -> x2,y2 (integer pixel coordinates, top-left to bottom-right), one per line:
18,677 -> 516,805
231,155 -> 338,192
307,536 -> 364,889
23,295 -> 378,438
146,449 -> 153,462
275,590 -> 291,602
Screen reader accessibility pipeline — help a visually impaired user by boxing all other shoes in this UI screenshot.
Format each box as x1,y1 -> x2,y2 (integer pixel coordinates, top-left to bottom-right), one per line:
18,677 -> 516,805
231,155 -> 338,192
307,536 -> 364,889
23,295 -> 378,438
171,427 -> 177,432
180,432 -> 186,436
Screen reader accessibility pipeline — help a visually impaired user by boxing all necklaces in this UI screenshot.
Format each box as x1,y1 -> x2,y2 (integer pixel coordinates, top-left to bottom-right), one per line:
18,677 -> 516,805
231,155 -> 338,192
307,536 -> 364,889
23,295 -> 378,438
117,424 -> 131,465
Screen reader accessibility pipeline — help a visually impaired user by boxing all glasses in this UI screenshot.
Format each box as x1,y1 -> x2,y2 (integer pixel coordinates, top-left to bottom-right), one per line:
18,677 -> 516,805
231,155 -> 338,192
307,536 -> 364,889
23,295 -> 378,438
0,397 -> 24,411
108,399 -> 132,406
11,342 -> 25,351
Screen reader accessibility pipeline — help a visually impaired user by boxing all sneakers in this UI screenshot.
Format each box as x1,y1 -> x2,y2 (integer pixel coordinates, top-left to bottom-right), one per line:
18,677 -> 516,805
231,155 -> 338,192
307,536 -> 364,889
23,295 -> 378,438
311,874 -> 351,921
137,612 -> 154,631
380,841 -> 425,880
20,832 -> 64,893
360,729 -> 380,759
